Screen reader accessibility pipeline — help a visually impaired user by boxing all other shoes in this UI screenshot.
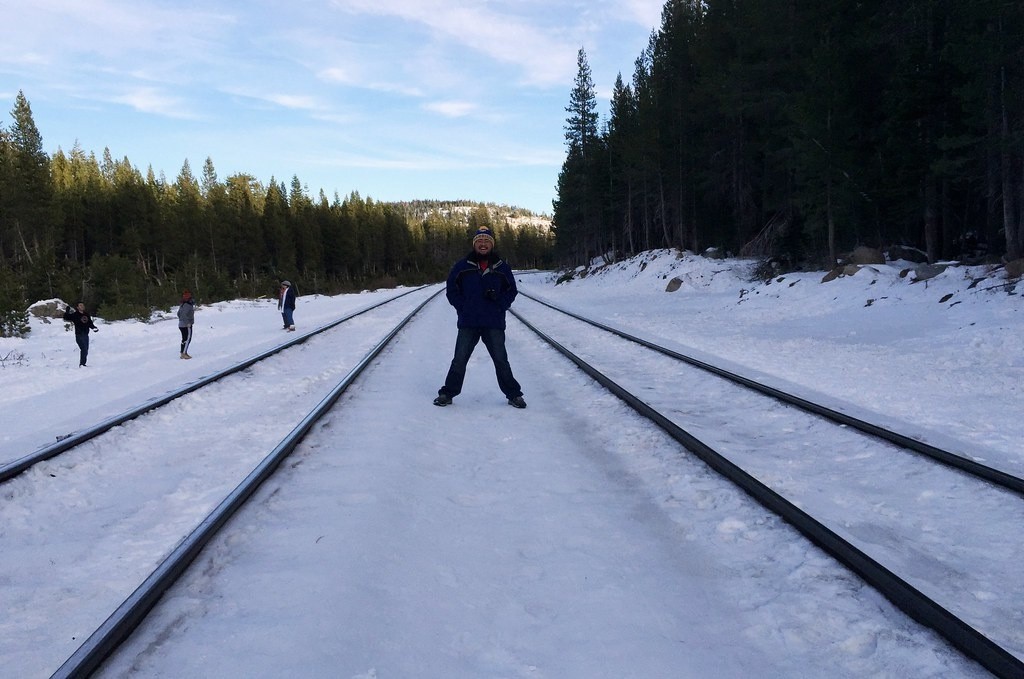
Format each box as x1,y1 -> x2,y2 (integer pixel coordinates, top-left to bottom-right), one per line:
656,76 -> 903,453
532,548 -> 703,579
434,392 -> 453,405
290,328 -> 295,331
180,353 -> 191,359
79,362 -> 87,368
284,325 -> 289,329
508,396 -> 526,408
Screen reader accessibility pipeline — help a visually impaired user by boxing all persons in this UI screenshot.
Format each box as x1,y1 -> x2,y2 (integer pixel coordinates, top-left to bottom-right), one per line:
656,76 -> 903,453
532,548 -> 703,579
434,226 -> 526,408
64,302 -> 98,367
177,292 -> 194,359
277,280 -> 295,331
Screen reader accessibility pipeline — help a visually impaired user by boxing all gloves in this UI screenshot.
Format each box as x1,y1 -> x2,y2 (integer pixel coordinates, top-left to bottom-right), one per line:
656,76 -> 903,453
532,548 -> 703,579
66,306 -> 70,313
93,327 -> 98,332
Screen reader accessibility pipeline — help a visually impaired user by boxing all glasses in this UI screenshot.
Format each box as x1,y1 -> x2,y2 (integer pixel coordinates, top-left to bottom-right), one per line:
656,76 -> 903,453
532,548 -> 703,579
477,240 -> 490,244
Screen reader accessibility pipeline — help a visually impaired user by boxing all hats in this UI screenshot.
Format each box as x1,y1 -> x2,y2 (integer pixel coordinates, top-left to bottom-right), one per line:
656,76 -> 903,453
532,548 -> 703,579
281,281 -> 291,286
183,290 -> 191,299
472,226 -> 495,248
